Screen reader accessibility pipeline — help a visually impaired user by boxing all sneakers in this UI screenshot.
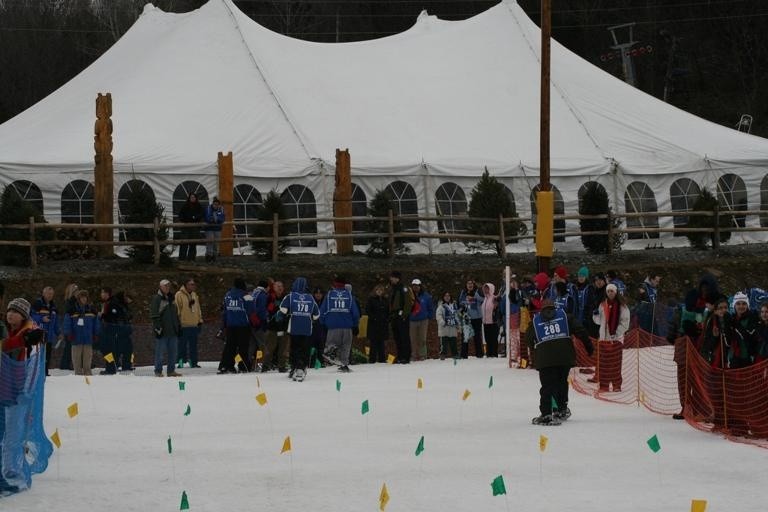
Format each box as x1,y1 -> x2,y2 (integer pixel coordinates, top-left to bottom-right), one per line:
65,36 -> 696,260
166,372 -> 183,377
532,415 -> 552,424
672,413 -> 685,419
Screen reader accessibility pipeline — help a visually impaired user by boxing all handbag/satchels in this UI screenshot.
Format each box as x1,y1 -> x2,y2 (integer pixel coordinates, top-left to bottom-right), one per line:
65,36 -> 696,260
269,310 -> 288,331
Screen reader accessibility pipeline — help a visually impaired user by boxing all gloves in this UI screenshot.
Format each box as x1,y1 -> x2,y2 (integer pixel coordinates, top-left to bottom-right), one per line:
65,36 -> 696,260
26,328 -> 48,345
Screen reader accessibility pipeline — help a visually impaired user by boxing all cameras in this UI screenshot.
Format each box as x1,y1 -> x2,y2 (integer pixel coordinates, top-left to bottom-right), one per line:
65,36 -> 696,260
188,299 -> 195,308
23,327 -> 48,351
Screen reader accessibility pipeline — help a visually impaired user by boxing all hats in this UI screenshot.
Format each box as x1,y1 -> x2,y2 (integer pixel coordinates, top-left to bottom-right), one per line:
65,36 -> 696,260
606,284 -> 618,294
733,290 -> 750,312
160,279 -> 170,287
593,272 -> 605,283
510,266 -> 589,283
412,279 -> 422,285
541,299 -> 555,309
76,290 -> 90,306
7,297 -> 31,319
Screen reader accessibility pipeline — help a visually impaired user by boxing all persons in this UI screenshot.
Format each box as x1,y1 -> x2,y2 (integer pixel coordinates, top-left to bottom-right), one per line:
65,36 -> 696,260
217,277 -> 290,374
457,279 -> 485,359
592,284 -> 630,393
178,192 -> 201,263
631,271 -> 681,339
306,282 -> 362,368
383,270 -> 416,364
364,284 -> 390,364
319,276 -> 360,373
497,267 -> 626,383
63,289 -> 100,375
29,286 -> 63,377
175,279 -> 204,368
149,279 -> 183,377
480,282 -> 500,357
436,291 -> 462,360
204,196 -> 225,264
97,287 -> 136,375
665,275 -> 766,439
60,283 -> 80,370
525,299 -> 577,426
1,298 -> 43,493
276,277 -> 320,382
409,279 -> 435,361
